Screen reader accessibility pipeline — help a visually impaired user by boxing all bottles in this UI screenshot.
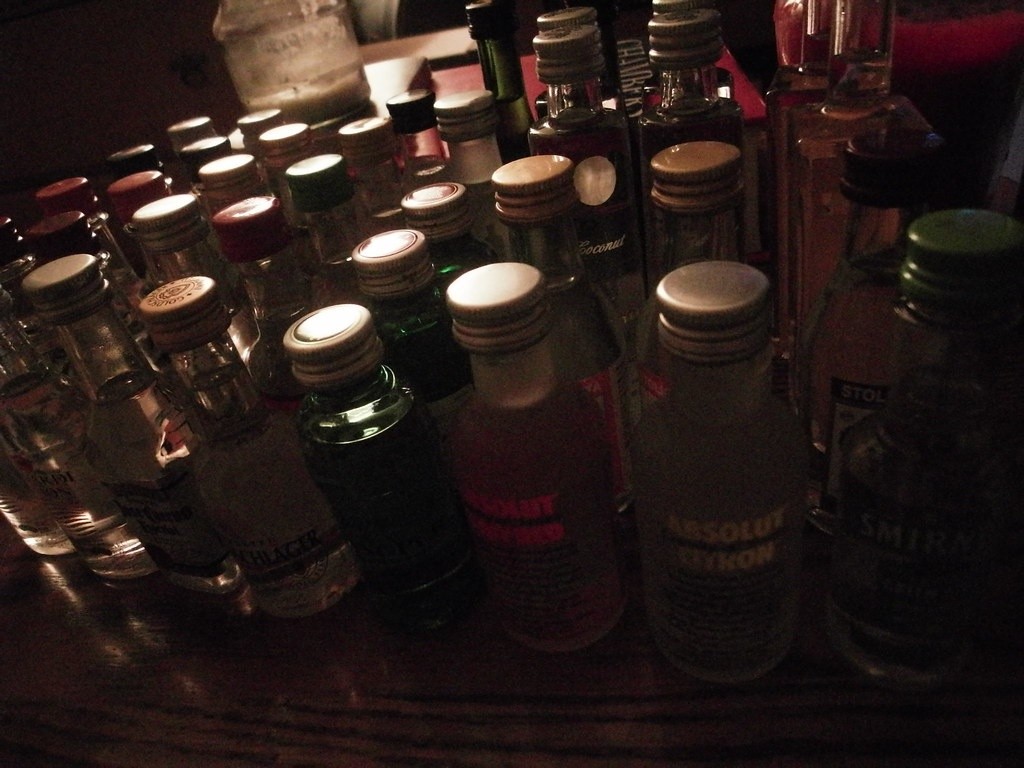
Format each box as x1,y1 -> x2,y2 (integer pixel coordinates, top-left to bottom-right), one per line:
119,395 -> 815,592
0,0 -> 1023,692
